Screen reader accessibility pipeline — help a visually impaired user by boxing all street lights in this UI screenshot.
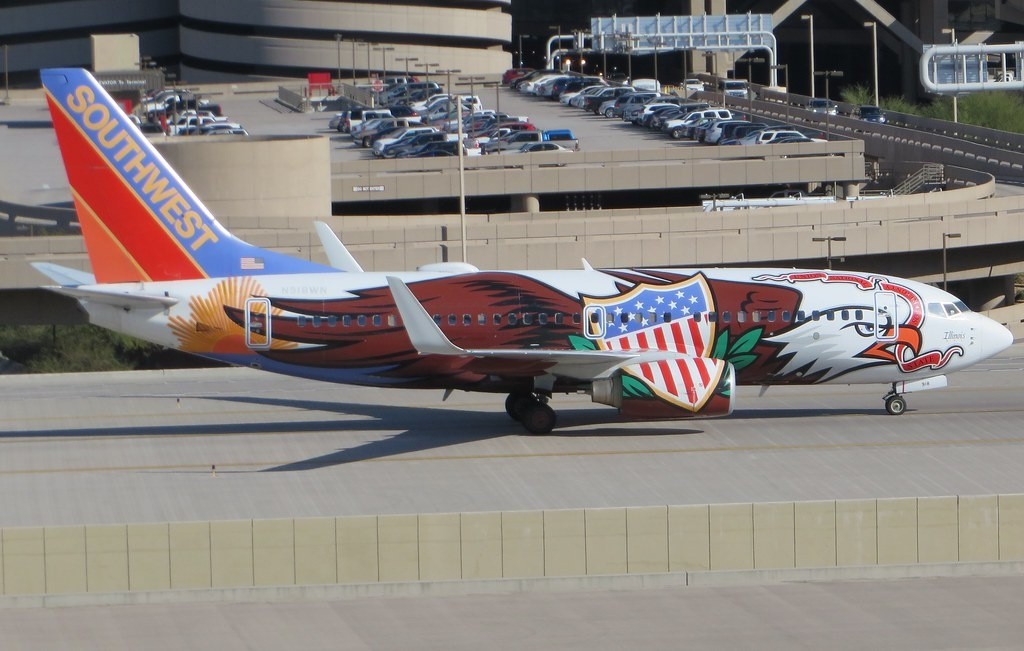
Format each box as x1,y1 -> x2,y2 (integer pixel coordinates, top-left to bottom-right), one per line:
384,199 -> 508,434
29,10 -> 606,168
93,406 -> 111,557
162,81 -> 188,135
674,46 -> 697,99
393,57 -> 419,105
335,33 -> 342,78
942,27 -> 958,124
458,76 -> 485,140
372,47 -> 396,105
771,65 -> 789,125
813,236 -> 846,270
943,233 -> 961,290
414,63 -> 440,126
703,54 -> 719,103
648,41 -> 667,91
435,69 -> 461,134
620,37 -> 639,81
815,72 -> 843,139
175,88 -> 200,135
801,14 -> 814,97
484,84 -> 509,153
864,22 -> 878,106
344,38 -> 360,82
549,26 -> 561,70
358,43 -> 379,82
520,35 -> 529,63
738,57 -> 765,120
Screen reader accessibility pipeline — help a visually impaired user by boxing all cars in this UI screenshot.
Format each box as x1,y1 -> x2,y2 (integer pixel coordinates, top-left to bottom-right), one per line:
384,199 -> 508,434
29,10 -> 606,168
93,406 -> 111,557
720,78 -> 748,97
805,98 -> 838,115
330,68 -> 831,169
850,104 -> 889,125
142,85 -> 249,137
680,79 -> 704,91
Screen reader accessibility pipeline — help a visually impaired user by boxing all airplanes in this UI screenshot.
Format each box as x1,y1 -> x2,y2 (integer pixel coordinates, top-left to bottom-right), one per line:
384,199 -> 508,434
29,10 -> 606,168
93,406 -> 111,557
30,66 -> 1014,434
